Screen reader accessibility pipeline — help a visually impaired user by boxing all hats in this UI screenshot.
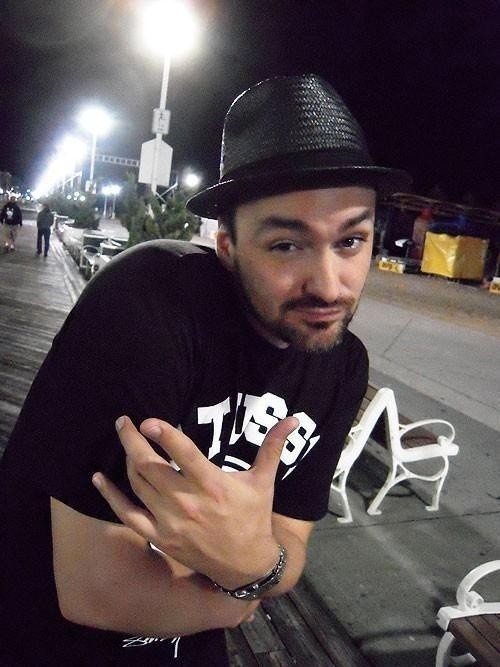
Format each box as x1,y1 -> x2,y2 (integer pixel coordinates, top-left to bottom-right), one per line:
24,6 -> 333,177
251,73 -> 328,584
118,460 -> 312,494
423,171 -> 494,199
185,72 -> 410,220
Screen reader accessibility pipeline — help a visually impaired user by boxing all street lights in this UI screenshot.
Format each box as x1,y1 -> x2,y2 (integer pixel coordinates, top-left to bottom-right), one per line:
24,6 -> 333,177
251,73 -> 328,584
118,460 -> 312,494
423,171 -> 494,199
140,8 -> 199,194
35,107 -> 120,220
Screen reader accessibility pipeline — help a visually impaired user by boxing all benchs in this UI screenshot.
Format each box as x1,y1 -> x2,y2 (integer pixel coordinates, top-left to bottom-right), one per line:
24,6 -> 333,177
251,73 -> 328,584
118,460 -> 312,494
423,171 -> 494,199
363,387 -> 460,516
328,380 -> 390,523
79,238 -> 124,276
435,559 -> 500,667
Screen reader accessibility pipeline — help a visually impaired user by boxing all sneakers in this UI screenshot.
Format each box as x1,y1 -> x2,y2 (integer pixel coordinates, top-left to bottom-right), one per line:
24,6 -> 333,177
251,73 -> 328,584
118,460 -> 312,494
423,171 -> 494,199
5,242 -> 48,257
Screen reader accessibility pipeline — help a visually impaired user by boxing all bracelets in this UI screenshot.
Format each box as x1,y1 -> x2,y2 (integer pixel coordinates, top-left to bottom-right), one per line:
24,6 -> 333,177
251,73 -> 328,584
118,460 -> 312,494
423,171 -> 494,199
210,539 -> 290,602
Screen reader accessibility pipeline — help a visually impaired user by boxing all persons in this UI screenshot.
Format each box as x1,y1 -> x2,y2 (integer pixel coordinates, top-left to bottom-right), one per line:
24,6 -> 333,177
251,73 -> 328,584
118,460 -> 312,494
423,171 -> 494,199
36,200 -> 54,257
1,73 -> 413,666
0,191 -> 23,249
